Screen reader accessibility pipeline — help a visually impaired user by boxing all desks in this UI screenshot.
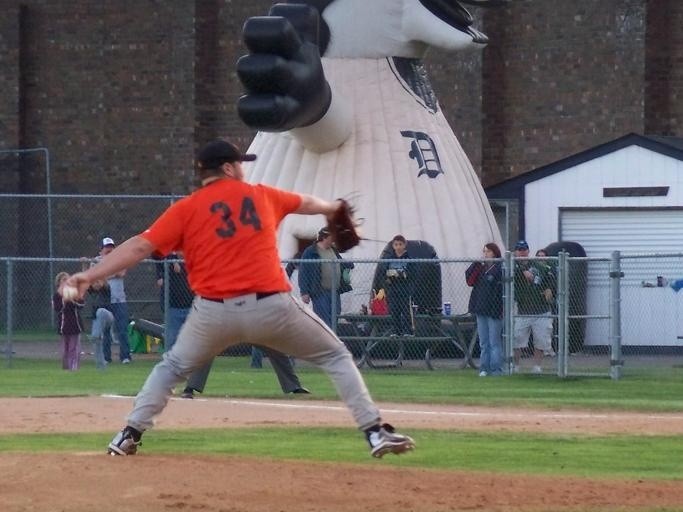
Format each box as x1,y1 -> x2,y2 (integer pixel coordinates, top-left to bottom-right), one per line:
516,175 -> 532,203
337,313 -> 478,371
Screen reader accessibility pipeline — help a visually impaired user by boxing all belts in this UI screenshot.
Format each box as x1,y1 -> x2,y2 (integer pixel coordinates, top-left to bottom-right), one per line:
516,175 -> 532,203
201,292 -> 279,303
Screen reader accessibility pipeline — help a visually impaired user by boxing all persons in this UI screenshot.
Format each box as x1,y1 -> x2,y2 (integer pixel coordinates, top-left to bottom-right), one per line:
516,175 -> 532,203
52,270 -> 86,373
510,239 -> 557,374
81,257 -> 117,371
534,249 -> 556,358
298,227 -> 354,335
180,346 -> 311,399
378,235 -> 418,339
153,247 -> 194,354
95,237 -> 132,364
56,139 -> 414,460
464,242 -> 504,377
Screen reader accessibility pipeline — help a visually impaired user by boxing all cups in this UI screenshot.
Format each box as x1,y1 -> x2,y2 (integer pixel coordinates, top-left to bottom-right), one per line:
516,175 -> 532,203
444,302 -> 451,316
411,301 -> 419,317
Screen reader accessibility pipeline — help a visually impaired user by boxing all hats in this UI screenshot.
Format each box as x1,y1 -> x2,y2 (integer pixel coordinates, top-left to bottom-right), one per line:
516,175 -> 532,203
513,240 -> 529,250
100,237 -> 115,248
318,227 -> 330,235
197,140 -> 256,169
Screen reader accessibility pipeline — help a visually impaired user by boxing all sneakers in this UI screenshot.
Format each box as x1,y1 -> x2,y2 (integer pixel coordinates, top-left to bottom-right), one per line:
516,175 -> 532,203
404,331 -> 415,337
480,369 -> 487,376
551,352 -> 556,358
122,358 -> 130,364
294,388 -> 312,394
107,426 -> 142,456
530,365 -> 541,373
544,352 -> 550,357
513,365 -> 521,374
181,392 -> 193,399
390,333 -> 402,337
364,424 -> 416,458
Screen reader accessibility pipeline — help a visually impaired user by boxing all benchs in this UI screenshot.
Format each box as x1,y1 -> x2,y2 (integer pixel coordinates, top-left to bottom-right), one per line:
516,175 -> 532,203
338,335 -> 455,371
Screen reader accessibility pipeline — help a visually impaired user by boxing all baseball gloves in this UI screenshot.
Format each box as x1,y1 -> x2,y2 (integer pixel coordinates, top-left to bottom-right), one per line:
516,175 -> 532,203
327,199 -> 359,253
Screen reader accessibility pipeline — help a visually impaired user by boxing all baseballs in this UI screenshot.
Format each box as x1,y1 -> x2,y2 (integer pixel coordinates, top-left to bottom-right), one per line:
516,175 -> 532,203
61,285 -> 77,299
